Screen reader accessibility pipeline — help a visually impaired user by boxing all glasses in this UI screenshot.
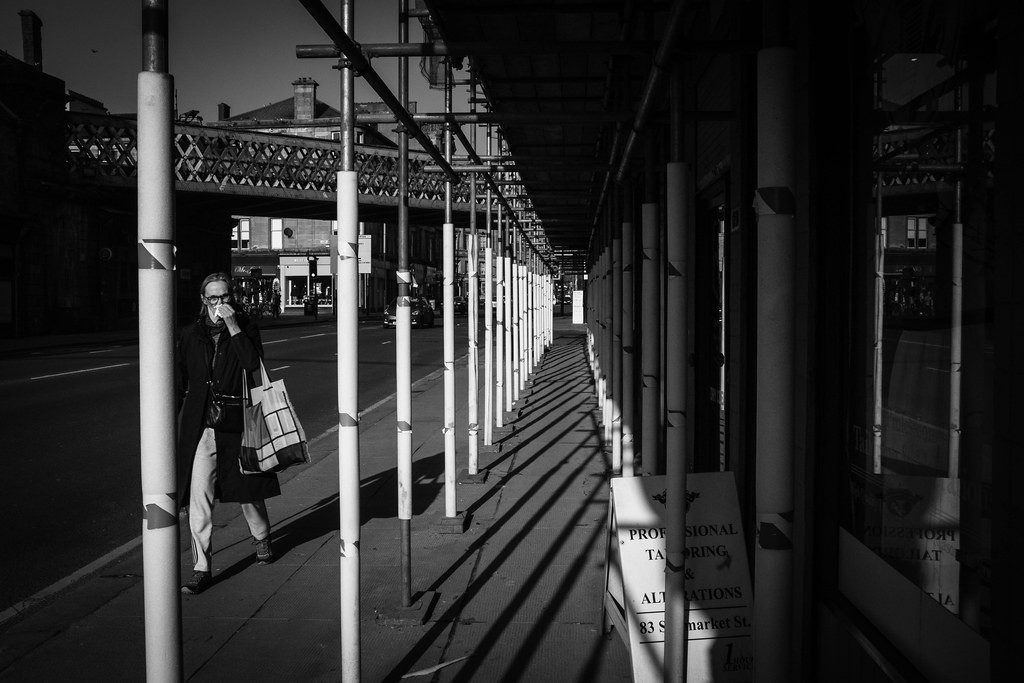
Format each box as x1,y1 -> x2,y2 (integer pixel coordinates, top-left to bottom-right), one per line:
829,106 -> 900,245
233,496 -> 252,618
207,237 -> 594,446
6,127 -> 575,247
202,293 -> 231,306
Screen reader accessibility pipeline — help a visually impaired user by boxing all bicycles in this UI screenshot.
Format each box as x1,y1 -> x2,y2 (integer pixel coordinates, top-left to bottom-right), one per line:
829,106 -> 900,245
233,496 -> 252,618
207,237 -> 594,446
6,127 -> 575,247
245,303 -> 282,319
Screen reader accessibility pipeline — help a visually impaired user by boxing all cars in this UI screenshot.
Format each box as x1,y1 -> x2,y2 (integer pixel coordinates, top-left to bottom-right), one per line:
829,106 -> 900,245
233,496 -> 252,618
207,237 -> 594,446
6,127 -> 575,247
484,296 -> 512,309
441,297 -> 467,316
384,296 -> 435,330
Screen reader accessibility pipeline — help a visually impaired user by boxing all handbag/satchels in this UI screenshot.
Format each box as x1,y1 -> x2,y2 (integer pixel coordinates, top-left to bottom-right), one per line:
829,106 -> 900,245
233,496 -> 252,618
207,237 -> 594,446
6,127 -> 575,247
236,355 -> 312,476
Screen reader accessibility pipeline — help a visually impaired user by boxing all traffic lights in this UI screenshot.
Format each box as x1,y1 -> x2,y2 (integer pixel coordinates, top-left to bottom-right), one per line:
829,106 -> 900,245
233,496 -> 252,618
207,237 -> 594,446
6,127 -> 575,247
251,269 -> 261,278
251,278 -> 258,285
308,260 -> 317,279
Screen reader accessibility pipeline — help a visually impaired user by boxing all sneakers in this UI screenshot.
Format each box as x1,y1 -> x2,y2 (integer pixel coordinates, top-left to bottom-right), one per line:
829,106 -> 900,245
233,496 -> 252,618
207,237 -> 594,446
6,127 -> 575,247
251,538 -> 275,566
181,570 -> 214,595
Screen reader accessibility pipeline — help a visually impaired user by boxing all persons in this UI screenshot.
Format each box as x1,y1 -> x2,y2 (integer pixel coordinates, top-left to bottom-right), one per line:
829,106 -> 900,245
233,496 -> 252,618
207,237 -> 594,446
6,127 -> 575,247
174,273 -> 281,594
271,289 -> 280,319
